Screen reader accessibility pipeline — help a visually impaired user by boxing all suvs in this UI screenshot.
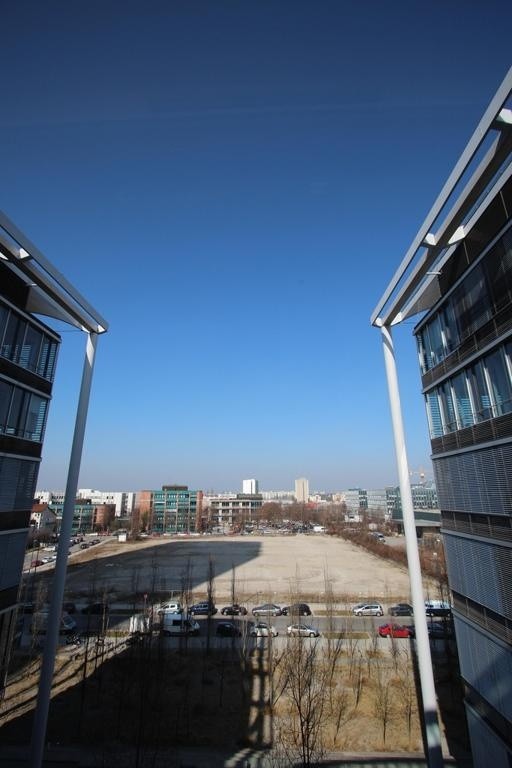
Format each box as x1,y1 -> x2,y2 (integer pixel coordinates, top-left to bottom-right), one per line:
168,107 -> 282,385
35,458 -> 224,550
189,603 -> 217,615
157,604 -> 181,615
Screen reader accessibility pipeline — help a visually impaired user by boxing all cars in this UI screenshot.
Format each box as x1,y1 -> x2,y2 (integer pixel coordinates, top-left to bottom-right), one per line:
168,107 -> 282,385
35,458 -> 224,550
249,624 -> 278,637
391,606 -> 411,616
379,623 -> 413,638
63,602 -> 76,614
353,604 -> 383,617
283,604 -> 311,616
428,623 -> 447,640
426,600 -> 451,611
374,532 -> 384,542
287,624 -> 318,638
252,604 -> 282,617
31,536 -> 101,569
221,606 -> 248,616
81,603 -> 108,616
216,622 -> 241,638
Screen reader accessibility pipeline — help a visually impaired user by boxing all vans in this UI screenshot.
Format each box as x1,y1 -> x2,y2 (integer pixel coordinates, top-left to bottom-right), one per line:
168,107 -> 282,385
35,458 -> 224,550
30,610 -> 78,635
162,614 -> 201,637
